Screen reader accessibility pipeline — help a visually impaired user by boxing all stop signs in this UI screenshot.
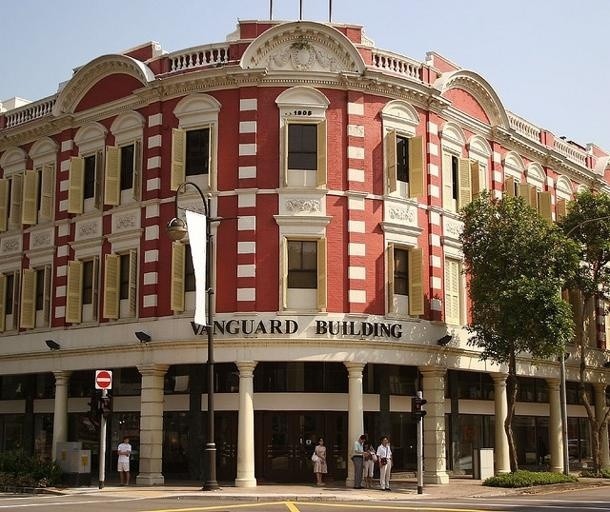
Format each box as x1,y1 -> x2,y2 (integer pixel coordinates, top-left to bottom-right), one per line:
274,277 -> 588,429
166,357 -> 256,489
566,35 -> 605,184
94,369 -> 112,391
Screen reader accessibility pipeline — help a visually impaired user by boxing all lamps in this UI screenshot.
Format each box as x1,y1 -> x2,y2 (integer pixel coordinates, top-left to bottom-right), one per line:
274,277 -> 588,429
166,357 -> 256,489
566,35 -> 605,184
605,361 -> 610,368
438,335 -> 453,345
45,340 -> 60,351
136,332 -> 151,342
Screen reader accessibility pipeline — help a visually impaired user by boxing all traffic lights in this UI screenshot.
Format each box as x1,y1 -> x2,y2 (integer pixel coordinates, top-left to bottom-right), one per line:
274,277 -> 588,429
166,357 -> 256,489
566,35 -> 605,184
410,396 -> 428,423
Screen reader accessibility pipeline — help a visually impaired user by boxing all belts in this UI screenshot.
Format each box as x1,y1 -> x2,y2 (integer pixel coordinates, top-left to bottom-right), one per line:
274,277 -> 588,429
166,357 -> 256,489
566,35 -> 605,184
353,456 -> 362,457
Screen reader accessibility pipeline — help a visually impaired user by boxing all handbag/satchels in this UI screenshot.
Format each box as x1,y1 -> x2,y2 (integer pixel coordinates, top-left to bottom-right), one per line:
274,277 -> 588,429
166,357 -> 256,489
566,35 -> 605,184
311,452 -> 319,462
381,458 -> 387,465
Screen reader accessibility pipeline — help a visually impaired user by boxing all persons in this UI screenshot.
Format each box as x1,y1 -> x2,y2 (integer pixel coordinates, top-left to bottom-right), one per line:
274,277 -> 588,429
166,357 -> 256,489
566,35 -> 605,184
350,435 -> 393,491
314,438 -> 328,486
117,436 -> 132,485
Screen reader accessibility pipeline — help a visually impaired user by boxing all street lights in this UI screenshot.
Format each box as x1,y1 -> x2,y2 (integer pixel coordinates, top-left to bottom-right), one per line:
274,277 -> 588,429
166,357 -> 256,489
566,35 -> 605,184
161,178 -> 222,492
